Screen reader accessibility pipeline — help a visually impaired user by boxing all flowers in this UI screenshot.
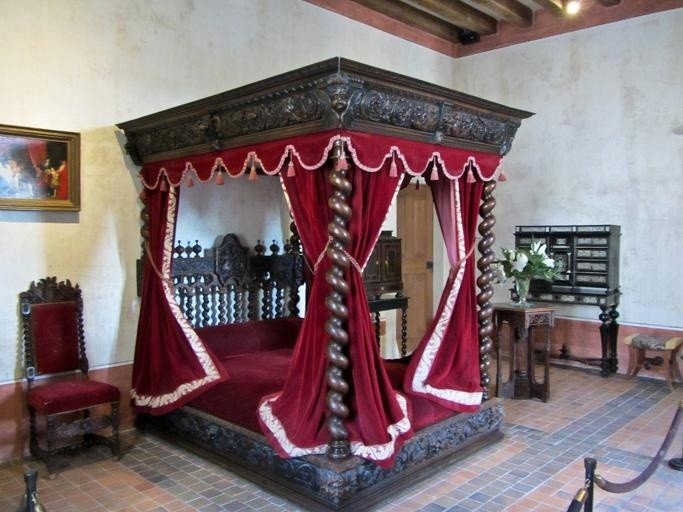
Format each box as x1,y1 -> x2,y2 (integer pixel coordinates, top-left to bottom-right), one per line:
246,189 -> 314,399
490,232 -> 562,286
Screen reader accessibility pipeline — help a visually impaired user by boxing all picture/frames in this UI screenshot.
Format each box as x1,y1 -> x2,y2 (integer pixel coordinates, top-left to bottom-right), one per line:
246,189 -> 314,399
0,122 -> 86,211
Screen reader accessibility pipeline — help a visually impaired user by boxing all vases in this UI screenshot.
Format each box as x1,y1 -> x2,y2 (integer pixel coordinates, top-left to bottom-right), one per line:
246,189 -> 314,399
515,278 -> 531,306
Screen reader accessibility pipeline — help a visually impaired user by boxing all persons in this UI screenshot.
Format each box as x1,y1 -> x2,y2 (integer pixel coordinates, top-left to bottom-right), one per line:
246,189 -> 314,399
0,153 -> 68,201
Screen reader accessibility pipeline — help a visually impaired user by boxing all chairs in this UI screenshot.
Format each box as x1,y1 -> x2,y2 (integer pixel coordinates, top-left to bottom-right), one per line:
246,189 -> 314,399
18,275 -> 120,481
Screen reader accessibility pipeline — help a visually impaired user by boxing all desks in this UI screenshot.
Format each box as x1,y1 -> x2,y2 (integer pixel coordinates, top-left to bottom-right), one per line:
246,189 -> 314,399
366,294 -> 410,359
490,303 -> 560,403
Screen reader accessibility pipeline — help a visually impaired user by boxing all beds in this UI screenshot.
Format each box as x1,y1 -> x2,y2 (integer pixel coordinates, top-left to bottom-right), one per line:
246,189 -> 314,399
114,57 -> 536,512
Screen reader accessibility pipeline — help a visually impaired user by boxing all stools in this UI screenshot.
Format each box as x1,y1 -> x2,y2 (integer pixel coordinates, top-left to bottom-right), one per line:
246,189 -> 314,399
624,333 -> 683,392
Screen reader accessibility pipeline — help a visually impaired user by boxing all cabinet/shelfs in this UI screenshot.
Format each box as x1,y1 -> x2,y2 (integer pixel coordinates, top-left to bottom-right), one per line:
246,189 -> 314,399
510,224 -> 623,377
361,230 -> 411,300
